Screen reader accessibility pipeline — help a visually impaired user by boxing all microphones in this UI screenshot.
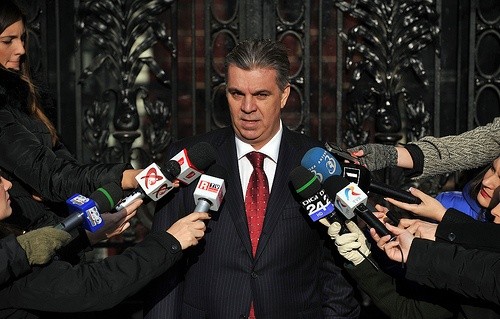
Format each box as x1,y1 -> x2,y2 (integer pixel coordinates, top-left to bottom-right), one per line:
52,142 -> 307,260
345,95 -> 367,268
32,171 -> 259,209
290,146 -> 422,269
54,141 -> 228,237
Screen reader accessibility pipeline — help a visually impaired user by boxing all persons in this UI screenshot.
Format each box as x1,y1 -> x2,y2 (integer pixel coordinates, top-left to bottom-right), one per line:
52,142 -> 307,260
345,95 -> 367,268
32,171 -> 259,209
131,37 -> 365,318
397,184 -> 500,252
0,169 -> 144,268
370,154 -> 500,238
0,224 -> 71,289
368,222 -> 500,306
384,187 -> 500,253
341,115 -> 500,181
0,169 -> 213,319
0,0 -> 181,215
316,216 -> 500,318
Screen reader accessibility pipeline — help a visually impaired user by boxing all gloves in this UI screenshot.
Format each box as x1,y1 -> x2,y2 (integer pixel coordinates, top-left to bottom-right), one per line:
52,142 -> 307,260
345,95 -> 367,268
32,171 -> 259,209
318,216 -> 371,266
16,225 -> 72,264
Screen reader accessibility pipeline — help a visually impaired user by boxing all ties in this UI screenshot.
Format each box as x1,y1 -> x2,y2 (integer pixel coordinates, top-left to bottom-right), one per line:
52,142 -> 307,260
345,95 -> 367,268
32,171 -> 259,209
244,151 -> 270,319
